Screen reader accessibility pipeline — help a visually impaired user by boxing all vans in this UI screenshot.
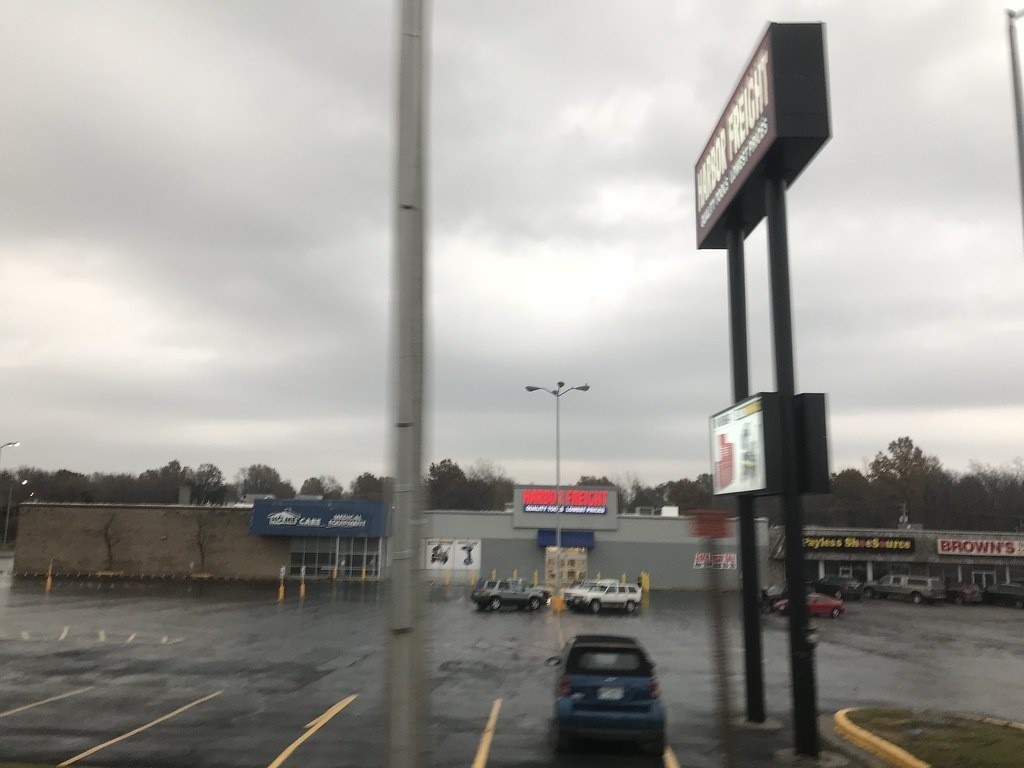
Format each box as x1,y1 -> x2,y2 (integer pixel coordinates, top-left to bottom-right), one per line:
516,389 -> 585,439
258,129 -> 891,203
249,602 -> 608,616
862,573 -> 946,604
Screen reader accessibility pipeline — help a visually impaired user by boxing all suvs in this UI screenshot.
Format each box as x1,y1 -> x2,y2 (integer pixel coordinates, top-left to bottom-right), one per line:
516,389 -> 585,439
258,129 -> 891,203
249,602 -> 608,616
573,582 -> 644,613
471,579 -> 549,612
563,577 -> 620,608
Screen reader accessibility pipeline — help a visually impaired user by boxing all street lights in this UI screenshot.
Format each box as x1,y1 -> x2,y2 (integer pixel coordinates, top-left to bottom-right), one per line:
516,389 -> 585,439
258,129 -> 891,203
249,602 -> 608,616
25,491 -> 34,502
524,378 -> 590,596
3,479 -> 28,544
1,441 -> 22,451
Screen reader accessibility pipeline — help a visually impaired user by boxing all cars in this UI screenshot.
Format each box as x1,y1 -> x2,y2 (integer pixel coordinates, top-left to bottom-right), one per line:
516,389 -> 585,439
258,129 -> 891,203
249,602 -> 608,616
947,582 -> 983,608
544,632 -> 668,753
980,581 -> 1024,611
773,592 -> 845,618
814,573 -> 866,600
